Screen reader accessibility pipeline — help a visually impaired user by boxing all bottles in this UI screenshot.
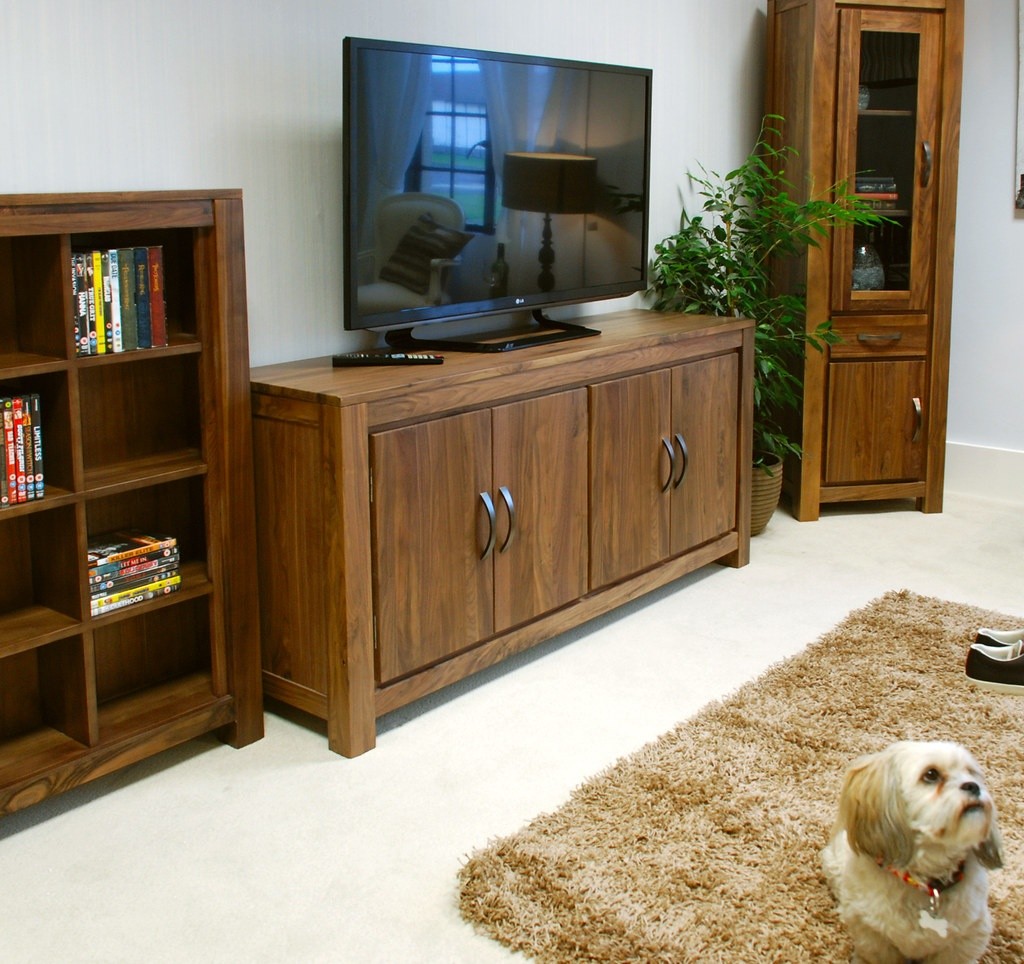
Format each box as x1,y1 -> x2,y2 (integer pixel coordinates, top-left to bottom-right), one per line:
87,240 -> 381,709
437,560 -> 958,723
489,243 -> 512,298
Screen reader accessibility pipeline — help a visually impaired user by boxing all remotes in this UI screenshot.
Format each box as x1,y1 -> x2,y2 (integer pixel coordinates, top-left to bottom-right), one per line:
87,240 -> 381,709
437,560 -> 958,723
332,353 -> 444,368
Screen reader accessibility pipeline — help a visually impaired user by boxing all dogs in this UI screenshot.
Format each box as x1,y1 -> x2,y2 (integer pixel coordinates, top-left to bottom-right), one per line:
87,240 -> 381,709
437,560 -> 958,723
819,739 -> 1006,964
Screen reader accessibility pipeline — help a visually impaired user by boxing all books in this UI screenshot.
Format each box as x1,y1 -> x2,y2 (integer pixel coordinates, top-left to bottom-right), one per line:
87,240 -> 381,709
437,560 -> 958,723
855,176 -> 899,209
88,528 -> 183,617
72,245 -> 169,357
0,394 -> 45,509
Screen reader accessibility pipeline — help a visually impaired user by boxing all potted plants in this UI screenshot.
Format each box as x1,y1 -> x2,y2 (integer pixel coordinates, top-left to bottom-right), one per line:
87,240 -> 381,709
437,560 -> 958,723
643,113 -> 903,536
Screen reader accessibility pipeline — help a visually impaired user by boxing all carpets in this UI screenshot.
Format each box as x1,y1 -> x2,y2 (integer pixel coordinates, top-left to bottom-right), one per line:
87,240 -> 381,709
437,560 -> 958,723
454,588 -> 1024,964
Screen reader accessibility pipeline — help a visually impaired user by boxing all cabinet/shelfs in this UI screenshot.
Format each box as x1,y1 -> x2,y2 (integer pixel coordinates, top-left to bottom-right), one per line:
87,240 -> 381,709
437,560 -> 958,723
250,309 -> 758,757
767,0 -> 964,524
0,189 -> 264,814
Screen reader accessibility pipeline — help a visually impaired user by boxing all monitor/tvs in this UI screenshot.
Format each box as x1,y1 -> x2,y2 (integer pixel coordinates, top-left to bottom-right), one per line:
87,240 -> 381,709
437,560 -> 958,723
341,36 -> 654,352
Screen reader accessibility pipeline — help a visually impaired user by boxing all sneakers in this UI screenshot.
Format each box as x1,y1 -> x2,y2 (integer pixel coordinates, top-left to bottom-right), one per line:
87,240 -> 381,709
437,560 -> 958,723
975,628 -> 1024,647
964,639 -> 1024,695
